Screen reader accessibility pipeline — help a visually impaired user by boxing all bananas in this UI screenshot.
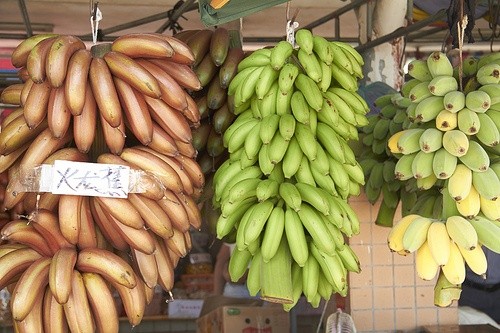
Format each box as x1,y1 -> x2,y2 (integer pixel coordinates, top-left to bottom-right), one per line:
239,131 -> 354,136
0,27 -> 499,333
